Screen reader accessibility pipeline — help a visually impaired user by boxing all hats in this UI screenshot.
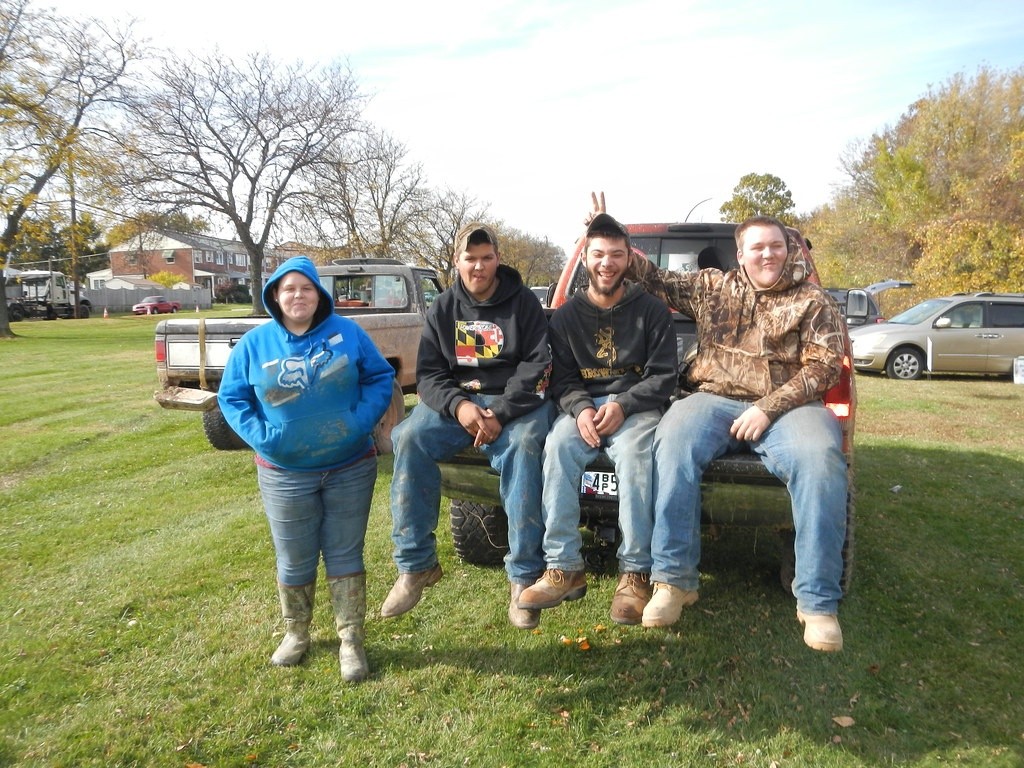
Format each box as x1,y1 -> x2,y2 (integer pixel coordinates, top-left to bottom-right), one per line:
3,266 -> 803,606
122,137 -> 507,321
457,222 -> 496,251
586,213 -> 629,238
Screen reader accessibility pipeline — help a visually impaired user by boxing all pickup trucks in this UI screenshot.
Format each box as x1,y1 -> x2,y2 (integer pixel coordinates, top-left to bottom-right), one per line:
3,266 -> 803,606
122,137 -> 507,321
132,295 -> 180,312
151,260 -> 455,455
417,226 -> 869,599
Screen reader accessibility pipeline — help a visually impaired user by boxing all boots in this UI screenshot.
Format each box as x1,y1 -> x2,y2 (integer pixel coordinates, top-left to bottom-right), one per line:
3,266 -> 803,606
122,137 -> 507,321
326,571 -> 369,683
270,575 -> 317,666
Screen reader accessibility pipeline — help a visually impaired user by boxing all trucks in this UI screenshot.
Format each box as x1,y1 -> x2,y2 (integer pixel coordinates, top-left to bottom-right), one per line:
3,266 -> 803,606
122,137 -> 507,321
3,267 -> 93,321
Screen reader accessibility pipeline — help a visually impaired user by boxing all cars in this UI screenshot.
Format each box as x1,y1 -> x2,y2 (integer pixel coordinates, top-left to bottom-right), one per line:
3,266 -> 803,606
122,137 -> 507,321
845,292 -> 1024,378
823,279 -> 914,330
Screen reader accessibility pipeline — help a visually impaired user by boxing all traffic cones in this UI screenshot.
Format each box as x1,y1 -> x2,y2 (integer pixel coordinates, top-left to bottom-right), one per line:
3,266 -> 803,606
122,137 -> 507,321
103,307 -> 111,319
195,304 -> 199,313
147,308 -> 151,315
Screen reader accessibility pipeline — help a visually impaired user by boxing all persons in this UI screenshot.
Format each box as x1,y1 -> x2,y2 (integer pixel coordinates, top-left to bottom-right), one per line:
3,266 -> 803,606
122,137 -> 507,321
217,257 -> 395,680
381,221 -> 558,628
584,191 -> 850,652
519,214 -> 678,624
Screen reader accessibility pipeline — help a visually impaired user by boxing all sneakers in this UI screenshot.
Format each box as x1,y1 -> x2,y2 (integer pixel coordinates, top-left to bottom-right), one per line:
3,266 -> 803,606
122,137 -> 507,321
509,580 -> 541,630
641,580 -> 700,627
517,567 -> 588,609
611,570 -> 653,625
796,609 -> 843,650
380,562 -> 444,618
